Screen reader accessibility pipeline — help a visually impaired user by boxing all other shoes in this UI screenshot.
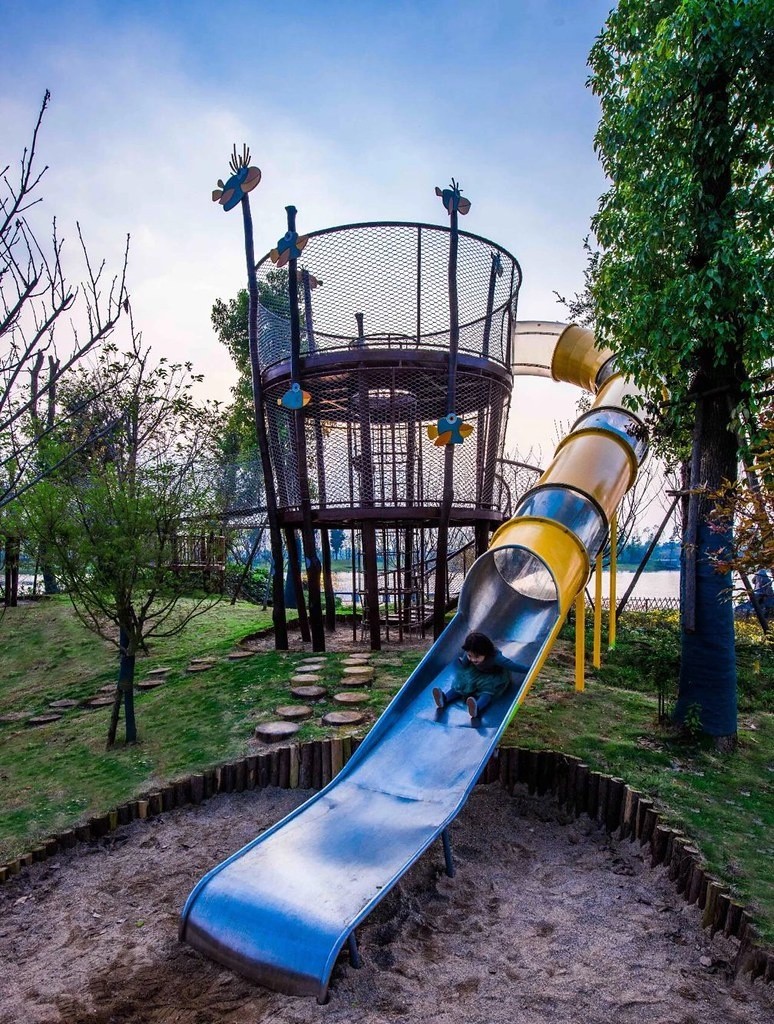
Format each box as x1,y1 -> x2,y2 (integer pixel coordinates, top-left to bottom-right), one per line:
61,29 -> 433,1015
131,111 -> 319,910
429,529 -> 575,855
433,687 -> 446,708
467,697 -> 479,718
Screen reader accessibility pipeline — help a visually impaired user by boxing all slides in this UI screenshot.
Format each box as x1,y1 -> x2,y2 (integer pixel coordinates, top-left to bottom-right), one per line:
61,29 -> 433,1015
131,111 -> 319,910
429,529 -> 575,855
178,320 -> 669,1000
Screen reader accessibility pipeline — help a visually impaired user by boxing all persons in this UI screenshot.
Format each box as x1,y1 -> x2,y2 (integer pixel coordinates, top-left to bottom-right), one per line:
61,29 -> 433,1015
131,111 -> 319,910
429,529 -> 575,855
433,633 -> 531,718
